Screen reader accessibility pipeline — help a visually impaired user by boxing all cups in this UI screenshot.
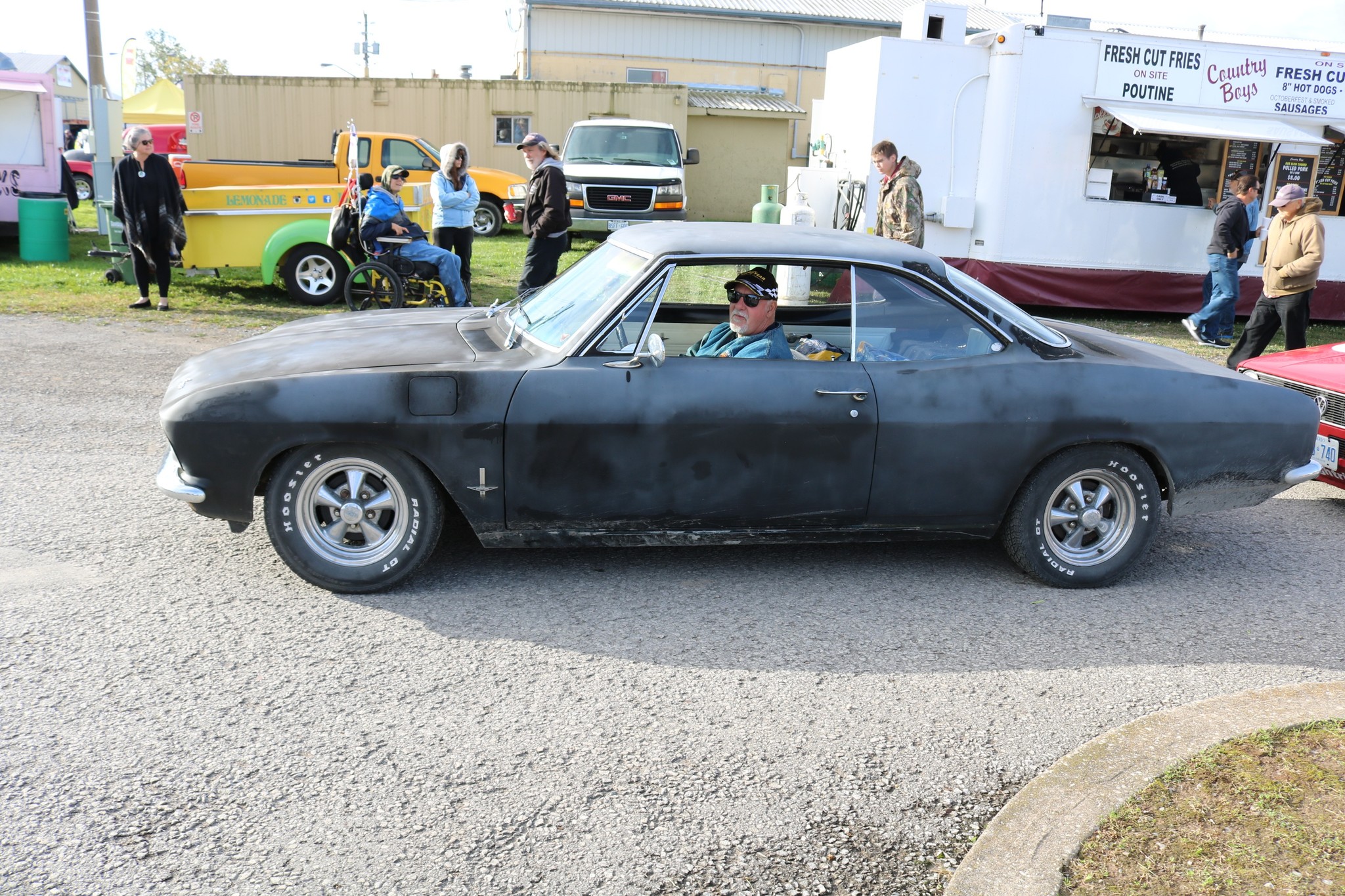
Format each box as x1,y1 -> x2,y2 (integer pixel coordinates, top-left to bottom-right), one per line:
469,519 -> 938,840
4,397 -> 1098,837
1166,188 -> 1171,194
503,201 -> 517,221
1109,144 -> 1118,153
1259,229 -> 1268,241
1262,217 -> 1272,229
1104,118 -> 1118,130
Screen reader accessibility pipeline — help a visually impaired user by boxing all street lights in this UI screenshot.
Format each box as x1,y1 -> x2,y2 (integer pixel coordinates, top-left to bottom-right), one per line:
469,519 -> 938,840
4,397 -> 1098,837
109,43 -> 181,91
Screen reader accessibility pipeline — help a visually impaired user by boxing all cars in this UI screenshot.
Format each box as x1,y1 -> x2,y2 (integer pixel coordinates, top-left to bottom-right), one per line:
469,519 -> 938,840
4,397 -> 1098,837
153,221 -> 1325,596
64,125 -> 188,200
1235,343 -> 1345,491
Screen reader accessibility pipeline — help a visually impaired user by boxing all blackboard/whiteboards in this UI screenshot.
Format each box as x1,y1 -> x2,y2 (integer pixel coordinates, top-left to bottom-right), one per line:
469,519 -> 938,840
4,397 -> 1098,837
1311,146 -> 1345,216
1216,139 -> 1263,205
1266,152 -> 1319,217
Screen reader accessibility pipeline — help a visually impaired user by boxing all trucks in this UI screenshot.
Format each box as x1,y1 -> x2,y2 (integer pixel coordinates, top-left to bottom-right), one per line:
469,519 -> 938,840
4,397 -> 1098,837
167,129 -> 529,238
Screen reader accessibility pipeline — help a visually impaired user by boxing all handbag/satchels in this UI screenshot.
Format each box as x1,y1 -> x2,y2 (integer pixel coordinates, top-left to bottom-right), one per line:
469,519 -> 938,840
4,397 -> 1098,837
326,178 -> 357,250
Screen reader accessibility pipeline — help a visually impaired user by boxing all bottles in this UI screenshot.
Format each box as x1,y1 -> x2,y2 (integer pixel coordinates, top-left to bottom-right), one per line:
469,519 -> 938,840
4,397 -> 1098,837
1151,167 -> 1157,179
1145,177 -> 1152,193
1157,166 -> 1164,180
1152,178 -> 1158,190
1144,164 -> 1152,180
1157,177 -> 1163,190
1162,177 -> 1167,190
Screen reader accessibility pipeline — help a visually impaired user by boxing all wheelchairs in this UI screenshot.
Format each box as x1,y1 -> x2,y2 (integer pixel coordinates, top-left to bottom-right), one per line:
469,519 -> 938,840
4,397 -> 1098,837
331,173 -> 473,312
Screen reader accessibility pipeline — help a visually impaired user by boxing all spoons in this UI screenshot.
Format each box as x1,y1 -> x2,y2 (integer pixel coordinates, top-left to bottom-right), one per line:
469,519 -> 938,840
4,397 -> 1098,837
1103,129 -> 1120,133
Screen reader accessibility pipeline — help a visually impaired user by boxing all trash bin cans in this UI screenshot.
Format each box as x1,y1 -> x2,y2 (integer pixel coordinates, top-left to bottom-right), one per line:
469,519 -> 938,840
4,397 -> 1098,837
17,191 -> 71,261
97,200 -> 136,284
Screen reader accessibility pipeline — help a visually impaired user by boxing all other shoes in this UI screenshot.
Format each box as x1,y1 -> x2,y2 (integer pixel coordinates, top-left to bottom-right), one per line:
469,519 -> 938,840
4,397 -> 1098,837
128,299 -> 151,308
157,302 -> 169,311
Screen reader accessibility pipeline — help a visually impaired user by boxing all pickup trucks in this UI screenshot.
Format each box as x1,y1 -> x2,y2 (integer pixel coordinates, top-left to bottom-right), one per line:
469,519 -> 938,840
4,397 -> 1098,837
548,118 -> 701,252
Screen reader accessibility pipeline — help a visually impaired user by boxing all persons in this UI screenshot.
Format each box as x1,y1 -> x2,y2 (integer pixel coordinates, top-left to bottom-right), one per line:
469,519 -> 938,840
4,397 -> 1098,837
63,129 -> 72,150
503,133 -> 573,302
112,125 -> 188,312
359,164 -> 472,308
870,142 -> 924,303
431,143 -> 479,308
1156,149 -> 1325,374
685,267 -> 791,359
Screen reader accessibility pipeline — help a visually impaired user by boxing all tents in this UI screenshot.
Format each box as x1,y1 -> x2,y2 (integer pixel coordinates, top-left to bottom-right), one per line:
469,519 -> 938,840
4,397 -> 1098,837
120,79 -> 189,129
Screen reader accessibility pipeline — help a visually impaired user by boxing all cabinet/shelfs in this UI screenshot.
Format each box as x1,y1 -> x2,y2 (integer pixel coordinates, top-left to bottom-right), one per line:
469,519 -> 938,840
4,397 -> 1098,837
1091,129 -> 1224,166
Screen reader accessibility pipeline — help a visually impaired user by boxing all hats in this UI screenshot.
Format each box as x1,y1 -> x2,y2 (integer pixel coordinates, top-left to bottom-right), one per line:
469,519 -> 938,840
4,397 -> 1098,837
1268,184 -> 1306,207
724,267 -> 778,300
1224,169 -> 1250,181
392,169 -> 409,177
518,119 -> 526,125
516,133 -> 547,150
499,130 -> 507,139
1154,140 -> 1169,160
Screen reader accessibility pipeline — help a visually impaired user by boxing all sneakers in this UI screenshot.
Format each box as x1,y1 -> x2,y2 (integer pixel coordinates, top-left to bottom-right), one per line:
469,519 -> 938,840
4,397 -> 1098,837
1181,317 -> 1207,343
1198,337 -> 1231,348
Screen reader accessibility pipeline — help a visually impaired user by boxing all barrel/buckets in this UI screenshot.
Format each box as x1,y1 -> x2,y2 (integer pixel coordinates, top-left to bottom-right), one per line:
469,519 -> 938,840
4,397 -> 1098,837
18,191 -> 69,261
168,154 -> 191,189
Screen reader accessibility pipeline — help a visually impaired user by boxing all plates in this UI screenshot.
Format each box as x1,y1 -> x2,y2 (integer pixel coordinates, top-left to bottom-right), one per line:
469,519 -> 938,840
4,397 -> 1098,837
1102,124 -> 1120,135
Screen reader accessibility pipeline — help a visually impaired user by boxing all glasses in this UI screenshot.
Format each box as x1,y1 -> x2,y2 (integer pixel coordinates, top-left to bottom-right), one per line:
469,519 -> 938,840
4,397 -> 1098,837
392,174 -> 404,180
727,288 -> 772,307
1253,186 -> 1262,193
872,158 -> 887,165
455,154 -> 464,159
1281,200 -> 1296,207
140,138 -> 153,145
